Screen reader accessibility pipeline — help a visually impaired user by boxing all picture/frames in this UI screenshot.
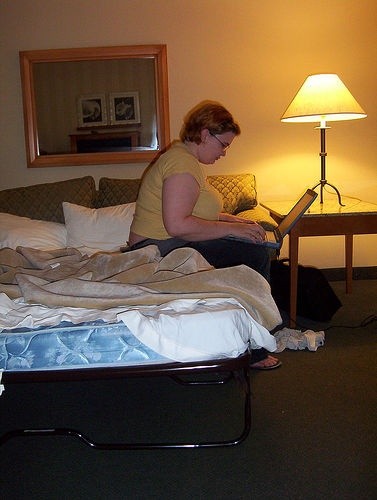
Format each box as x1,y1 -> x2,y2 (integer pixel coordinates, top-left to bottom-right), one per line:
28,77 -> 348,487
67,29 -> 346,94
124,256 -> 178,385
76,92 -> 107,127
109,91 -> 141,125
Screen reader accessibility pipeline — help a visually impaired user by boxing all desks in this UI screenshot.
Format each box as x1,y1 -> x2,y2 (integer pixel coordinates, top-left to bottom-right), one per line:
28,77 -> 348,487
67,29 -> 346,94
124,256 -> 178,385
259,198 -> 377,329
68,130 -> 141,154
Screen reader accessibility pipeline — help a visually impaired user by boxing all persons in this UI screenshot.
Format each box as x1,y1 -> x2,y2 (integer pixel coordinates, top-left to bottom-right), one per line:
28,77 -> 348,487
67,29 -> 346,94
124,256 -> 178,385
126,101 -> 281,370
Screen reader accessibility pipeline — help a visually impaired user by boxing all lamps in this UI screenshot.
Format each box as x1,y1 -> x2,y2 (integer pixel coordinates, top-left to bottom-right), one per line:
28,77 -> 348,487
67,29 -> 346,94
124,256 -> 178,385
280,73 -> 367,206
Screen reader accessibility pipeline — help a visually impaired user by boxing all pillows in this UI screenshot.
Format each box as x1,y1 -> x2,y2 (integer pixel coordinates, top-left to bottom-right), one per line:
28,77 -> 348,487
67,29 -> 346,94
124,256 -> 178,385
0,213 -> 67,250
0,176 -> 96,224
62,201 -> 136,250
97,174 -> 258,214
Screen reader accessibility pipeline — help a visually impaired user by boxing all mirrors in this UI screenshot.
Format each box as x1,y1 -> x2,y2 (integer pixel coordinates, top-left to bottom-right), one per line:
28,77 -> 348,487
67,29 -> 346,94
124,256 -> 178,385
19,44 -> 171,169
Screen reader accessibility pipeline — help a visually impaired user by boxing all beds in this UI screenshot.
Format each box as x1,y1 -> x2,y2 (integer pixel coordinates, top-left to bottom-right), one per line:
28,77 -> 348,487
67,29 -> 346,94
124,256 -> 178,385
0,246 -> 253,450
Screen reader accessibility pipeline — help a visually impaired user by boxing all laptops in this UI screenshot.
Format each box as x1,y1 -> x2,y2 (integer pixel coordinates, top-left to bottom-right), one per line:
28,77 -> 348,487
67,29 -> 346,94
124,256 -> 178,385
225,189 -> 318,248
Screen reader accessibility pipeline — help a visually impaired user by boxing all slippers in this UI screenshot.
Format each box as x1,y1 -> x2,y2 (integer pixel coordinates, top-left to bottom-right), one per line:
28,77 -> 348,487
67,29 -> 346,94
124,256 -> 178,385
248,356 -> 282,369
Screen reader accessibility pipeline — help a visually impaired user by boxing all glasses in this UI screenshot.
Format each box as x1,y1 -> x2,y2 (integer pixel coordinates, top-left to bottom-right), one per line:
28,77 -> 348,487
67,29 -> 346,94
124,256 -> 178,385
209,132 -> 230,151
